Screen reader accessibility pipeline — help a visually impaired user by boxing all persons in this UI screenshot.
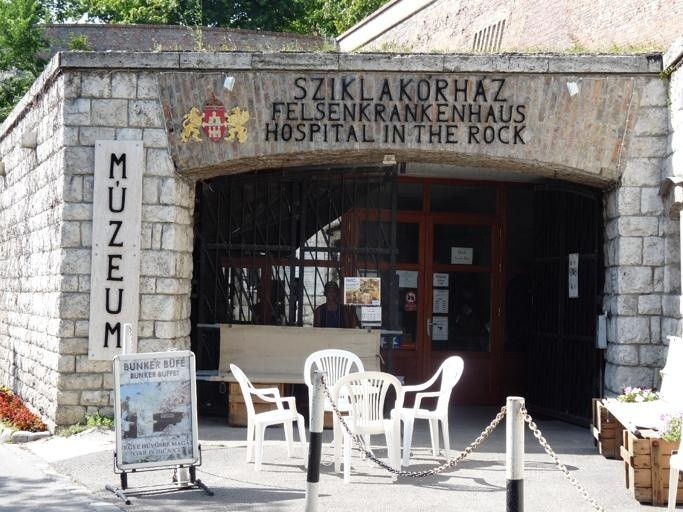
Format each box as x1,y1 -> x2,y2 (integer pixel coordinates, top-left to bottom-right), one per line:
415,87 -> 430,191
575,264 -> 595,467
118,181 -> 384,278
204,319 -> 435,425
313,280 -> 361,329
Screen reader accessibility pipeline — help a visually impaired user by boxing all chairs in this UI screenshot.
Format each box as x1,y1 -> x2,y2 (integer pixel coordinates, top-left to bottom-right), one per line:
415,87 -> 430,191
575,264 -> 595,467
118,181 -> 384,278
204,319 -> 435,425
228,347 -> 464,486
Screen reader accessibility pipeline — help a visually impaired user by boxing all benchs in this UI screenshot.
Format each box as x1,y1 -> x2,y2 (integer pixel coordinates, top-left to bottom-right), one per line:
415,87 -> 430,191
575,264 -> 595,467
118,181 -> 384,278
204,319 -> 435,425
591,336 -> 681,503
209,324 -> 381,429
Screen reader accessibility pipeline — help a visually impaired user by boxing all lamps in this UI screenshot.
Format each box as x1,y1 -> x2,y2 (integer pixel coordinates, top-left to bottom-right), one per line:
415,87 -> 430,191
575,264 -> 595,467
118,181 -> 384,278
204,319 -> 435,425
567,78 -> 580,97
222,72 -> 234,92
381,154 -> 396,166
